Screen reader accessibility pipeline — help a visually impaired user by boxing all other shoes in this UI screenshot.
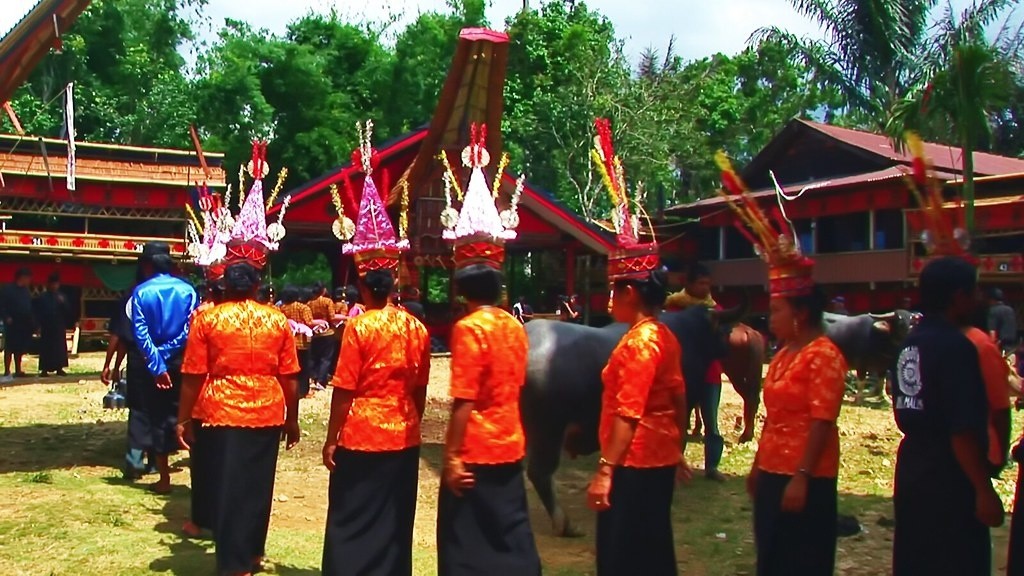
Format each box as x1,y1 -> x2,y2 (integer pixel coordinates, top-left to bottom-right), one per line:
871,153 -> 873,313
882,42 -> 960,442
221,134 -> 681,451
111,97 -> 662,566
57,370 -> 66,376
40,373 -> 50,377
183,521 -> 212,539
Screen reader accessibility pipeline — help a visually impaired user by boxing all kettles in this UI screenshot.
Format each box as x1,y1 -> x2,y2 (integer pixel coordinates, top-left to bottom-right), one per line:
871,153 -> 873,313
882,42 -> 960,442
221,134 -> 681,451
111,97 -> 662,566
111,367 -> 127,394
103,380 -> 126,409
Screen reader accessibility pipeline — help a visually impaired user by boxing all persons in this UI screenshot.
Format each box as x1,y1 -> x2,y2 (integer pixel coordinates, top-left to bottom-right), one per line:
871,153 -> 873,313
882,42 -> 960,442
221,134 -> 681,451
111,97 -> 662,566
746,261 -> 848,576
588,244 -> 694,576
558,294 -> 585,325
830,296 -> 849,315
512,296 -> 525,325
665,261 -> 725,483
0,267 -> 77,378
176,241 -> 300,576
101,241 -> 426,536
521,302 -> 534,323
322,251 -> 431,576
437,242 -> 542,576
893,255 -> 1024,576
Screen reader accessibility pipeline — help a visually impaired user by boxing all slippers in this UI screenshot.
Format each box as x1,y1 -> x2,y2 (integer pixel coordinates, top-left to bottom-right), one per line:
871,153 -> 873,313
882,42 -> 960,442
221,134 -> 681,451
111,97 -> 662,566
149,482 -> 171,494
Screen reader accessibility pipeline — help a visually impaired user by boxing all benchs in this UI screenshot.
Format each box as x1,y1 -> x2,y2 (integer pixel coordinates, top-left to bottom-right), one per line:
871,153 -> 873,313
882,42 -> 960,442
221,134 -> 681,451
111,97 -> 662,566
78,318 -> 111,343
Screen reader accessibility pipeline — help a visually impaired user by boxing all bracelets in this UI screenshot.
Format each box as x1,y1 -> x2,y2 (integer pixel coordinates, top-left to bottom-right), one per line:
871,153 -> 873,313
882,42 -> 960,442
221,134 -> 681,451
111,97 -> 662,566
598,458 -> 616,466
796,470 -> 812,479
177,418 -> 192,427
448,450 -> 464,454
325,441 -> 337,446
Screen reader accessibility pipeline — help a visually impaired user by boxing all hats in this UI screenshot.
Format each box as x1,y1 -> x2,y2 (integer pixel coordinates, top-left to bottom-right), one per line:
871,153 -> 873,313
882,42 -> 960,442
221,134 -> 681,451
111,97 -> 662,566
137,242 -> 170,261
195,139 -> 292,270
330,119 -> 410,277
186,182 -> 235,283
440,123 -> 526,277
715,149 -> 814,298
589,118 -> 661,284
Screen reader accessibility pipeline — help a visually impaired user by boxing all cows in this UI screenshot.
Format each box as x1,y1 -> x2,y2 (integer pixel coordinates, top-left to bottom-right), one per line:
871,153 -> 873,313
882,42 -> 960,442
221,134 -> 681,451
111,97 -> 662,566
828,309 -> 915,408
522,290 -> 751,537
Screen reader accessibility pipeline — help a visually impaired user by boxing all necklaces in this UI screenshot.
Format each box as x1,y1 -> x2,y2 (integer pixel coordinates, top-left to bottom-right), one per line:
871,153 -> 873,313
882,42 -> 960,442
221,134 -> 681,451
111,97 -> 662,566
622,317 -> 656,340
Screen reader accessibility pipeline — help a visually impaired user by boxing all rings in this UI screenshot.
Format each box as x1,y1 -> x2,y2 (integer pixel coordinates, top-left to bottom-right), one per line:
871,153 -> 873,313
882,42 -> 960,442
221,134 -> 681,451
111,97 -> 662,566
595,501 -> 601,505
